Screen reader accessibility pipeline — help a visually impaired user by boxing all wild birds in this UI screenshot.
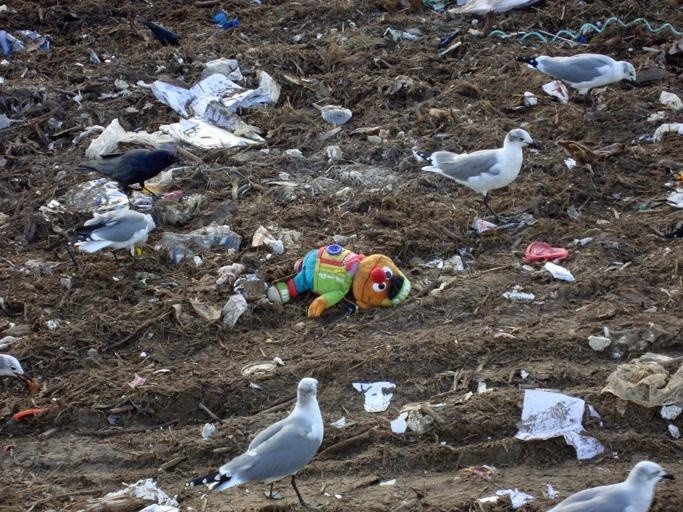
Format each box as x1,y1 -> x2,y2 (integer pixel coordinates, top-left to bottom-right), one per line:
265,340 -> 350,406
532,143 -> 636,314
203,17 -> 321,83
181,374 -> 326,510
43,191 -> 158,269
543,460 -> 676,512
513,50 -> 638,116
409,128 -> 543,226
75,145 -> 182,204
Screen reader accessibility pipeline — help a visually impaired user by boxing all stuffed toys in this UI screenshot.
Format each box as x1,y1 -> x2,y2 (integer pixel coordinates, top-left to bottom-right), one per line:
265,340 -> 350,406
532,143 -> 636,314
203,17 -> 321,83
267,242 -> 414,326
